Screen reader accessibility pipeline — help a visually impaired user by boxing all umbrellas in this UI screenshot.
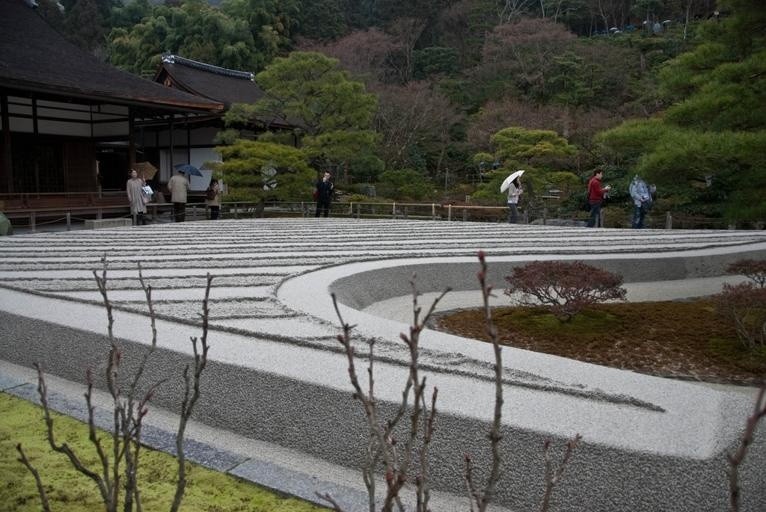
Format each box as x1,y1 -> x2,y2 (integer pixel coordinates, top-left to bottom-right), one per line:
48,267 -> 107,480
130,161 -> 159,181
174,164 -> 203,177
501,170 -> 525,193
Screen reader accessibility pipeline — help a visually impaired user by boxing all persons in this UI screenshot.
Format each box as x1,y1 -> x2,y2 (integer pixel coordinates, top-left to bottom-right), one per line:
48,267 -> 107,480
507,176 -> 523,224
584,169 -> 612,228
206,179 -> 221,220
167,170 -> 190,222
629,173 -> 657,229
313,171 -> 334,218
126,168 -> 146,226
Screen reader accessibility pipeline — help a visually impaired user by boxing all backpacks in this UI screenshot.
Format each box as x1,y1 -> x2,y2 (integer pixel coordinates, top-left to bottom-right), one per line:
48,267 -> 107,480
206,187 -> 217,201
